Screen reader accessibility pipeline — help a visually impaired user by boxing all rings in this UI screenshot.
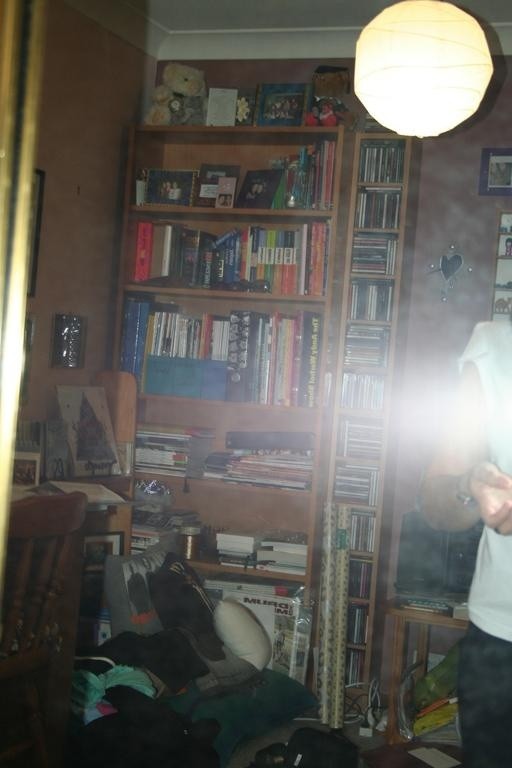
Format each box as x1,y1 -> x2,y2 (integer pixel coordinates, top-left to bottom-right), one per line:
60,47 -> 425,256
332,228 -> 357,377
492,525 -> 502,536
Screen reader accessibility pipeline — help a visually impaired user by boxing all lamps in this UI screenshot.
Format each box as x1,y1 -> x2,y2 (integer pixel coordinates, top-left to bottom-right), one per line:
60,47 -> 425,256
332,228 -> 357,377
353,0 -> 494,139
48,311 -> 88,369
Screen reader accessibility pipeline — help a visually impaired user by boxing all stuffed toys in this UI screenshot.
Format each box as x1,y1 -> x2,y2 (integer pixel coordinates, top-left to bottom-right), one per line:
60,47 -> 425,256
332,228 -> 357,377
143,59 -> 210,127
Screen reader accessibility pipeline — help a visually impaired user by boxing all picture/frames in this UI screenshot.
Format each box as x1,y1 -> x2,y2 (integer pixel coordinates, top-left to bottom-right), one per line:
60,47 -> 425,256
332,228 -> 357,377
252,81 -> 311,127
196,163 -> 240,206
488,209 -> 512,324
477,147 -> 512,195
26,169 -> 45,295
142,167 -> 199,207
12,452 -> 39,487
81,532 -> 124,572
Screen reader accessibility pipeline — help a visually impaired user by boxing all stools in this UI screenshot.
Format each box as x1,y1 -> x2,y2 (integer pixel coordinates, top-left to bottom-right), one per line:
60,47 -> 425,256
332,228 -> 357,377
360,740 -> 463,768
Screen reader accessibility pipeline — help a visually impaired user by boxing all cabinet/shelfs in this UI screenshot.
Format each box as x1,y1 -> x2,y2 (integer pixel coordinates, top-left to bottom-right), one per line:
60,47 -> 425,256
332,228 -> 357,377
312,132 -> 423,728
101,123 -> 353,693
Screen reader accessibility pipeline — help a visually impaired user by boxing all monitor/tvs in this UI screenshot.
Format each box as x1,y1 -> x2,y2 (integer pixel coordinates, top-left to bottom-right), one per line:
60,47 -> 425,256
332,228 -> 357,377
395,513 -> 483,614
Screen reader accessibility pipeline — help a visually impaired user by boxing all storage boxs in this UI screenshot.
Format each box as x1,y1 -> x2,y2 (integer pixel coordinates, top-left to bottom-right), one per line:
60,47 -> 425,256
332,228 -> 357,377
143,354 -> 229,400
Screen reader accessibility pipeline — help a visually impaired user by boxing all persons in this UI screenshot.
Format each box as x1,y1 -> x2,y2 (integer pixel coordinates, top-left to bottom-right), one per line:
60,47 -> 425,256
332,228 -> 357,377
425,317 -> 512,768
161,170 -> 265,207
266,95 -> 343,128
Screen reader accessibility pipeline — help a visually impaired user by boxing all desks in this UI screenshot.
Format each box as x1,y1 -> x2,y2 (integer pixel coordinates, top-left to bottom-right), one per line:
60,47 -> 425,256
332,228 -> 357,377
380,595 -> 471,735
9,482 -> 132,608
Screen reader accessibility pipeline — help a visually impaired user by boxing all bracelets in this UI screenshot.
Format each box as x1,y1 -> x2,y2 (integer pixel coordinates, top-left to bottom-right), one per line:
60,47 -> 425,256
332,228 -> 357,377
456,455 -> 484,505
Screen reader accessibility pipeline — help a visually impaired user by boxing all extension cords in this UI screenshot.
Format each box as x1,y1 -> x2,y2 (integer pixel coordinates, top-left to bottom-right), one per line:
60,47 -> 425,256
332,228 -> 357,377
359,710 -> 382,738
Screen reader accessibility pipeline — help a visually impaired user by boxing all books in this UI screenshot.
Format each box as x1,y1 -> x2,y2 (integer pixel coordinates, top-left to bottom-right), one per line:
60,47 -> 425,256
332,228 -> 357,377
131,509 -> 308,575
330,145 -> 405,685
53,382 -> 120,478
126,217 -> 331,297
269,139 -> 336,210
203,580 -> 312,687
124,299 -> 323,424
132,426 -> 315,490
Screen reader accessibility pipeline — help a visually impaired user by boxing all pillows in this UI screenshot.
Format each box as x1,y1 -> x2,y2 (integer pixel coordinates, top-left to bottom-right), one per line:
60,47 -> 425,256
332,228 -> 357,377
187,667 -> 318,767
211,599 -> 272,668
145,551 -> 225,660
100,543 -> 169,667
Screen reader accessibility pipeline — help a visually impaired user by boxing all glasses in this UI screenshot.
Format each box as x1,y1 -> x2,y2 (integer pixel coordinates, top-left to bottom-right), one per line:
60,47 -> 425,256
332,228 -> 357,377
210,278 -> 269,292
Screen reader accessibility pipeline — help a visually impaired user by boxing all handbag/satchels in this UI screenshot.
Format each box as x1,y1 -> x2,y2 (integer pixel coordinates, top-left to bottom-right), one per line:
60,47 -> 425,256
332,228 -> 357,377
149,552 -> 213,637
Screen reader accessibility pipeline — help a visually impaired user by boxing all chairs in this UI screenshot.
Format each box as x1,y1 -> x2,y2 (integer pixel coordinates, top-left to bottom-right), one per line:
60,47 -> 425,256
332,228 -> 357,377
0,489 -> 88,768
